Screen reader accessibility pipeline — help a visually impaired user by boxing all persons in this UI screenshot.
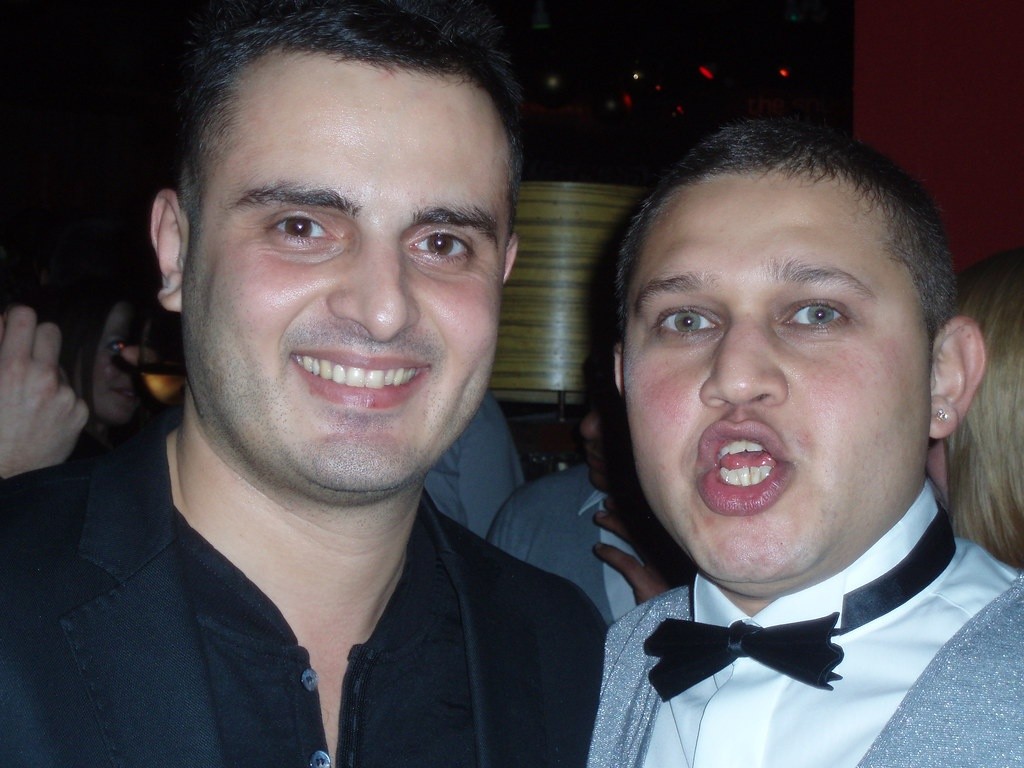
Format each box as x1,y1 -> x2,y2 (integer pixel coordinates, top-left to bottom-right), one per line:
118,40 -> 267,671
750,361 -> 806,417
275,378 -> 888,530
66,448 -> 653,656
587,122 -> 1024,768
1,0 -> 606,768
0,251 -> 1024,624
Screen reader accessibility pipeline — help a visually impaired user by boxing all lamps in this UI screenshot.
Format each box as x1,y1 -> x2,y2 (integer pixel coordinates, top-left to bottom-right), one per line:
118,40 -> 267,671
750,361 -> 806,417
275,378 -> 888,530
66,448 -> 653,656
488,181 -> 648,454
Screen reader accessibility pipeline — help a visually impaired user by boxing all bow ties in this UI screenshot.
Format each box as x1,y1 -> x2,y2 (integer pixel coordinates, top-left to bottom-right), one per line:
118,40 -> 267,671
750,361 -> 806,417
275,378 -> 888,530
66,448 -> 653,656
643,501 -> 957,704
